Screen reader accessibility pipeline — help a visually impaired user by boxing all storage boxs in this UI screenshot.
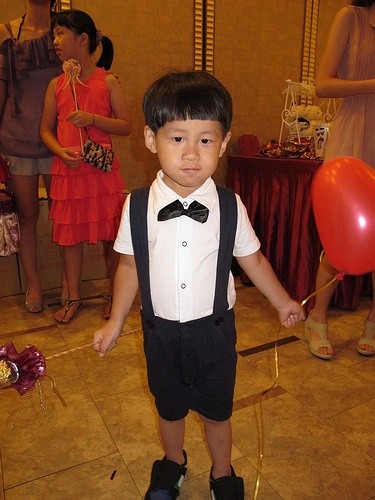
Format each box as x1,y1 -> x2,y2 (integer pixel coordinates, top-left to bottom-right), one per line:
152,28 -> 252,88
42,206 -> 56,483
0,187 -> 110,298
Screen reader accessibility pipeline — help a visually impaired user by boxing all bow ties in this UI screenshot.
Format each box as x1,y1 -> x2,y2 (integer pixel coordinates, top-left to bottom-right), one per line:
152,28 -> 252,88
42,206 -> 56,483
156,199 -> 209,224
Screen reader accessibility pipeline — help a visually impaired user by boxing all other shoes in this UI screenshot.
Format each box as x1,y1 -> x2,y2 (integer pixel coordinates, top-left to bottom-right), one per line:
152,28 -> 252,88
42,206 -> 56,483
209,462 -> 244,500
144,449 -> 188,500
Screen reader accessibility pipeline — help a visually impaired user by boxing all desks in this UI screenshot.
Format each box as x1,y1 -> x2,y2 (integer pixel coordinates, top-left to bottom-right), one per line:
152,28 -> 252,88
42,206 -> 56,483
226,155 -> 363,319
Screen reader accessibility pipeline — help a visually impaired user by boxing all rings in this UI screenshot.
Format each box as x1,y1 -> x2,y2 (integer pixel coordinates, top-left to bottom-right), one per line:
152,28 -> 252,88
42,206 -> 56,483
72,121 -> 78,126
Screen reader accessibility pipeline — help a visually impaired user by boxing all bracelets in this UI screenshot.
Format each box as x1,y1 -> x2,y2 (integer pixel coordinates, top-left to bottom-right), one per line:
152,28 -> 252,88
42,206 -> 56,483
92,113 -> 96,126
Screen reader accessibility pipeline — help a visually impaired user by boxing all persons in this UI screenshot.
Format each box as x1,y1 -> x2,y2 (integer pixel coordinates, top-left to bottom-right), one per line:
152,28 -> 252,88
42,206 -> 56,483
92,66 -> 306,500
0,0 -> 122,313
39,8 -> 134,324
305,1 -> 375,359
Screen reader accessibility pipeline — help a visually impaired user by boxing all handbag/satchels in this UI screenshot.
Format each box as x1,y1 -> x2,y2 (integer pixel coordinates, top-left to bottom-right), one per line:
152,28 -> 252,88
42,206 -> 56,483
80,140 -> 114,172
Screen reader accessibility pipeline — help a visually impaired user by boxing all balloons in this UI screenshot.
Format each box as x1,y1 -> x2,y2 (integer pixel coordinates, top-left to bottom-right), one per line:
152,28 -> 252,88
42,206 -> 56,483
310,156 -> 375,276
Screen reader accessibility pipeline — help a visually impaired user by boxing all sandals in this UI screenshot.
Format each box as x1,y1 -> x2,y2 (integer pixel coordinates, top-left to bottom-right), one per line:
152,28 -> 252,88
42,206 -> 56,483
25,289 -> 44,312
55,298 -> 83,324
58,295 -> 70,305
104,294 -> 114,320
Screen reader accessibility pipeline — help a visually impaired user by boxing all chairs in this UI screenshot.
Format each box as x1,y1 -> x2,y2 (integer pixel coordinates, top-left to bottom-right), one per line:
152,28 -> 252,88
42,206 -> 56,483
239,134 -> 260,154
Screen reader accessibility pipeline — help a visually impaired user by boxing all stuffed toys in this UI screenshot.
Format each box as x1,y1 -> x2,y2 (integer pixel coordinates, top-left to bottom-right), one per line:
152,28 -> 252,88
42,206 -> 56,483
303,103 -> 327,136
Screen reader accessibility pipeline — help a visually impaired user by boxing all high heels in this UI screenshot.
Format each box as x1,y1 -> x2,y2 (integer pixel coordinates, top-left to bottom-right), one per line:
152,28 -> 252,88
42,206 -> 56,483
358,321 -> 375,355
305,316 -> 334,358
